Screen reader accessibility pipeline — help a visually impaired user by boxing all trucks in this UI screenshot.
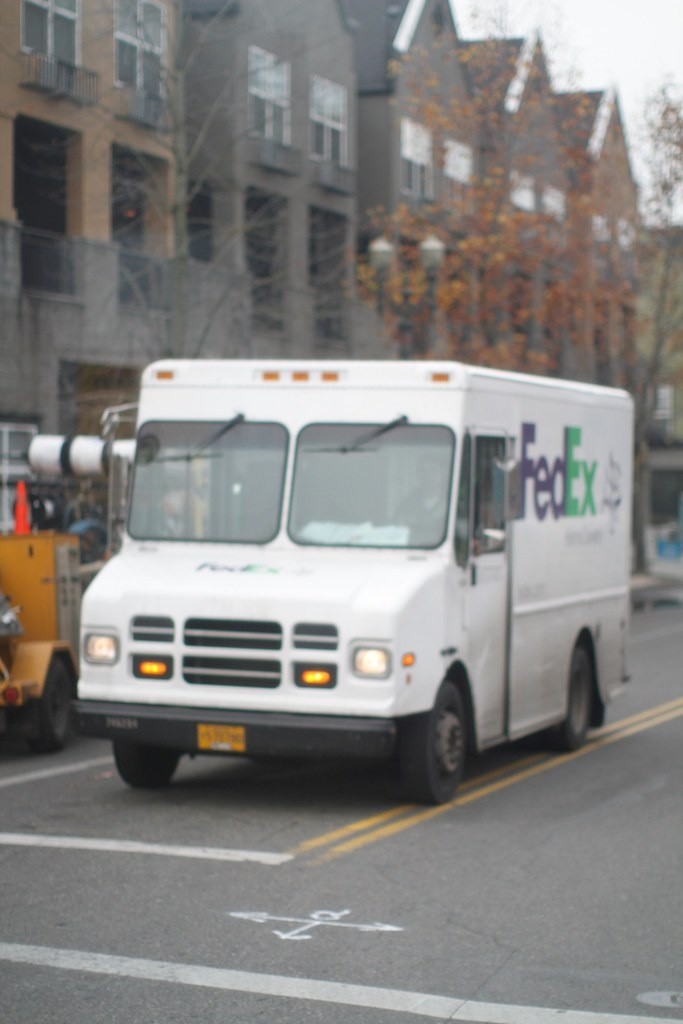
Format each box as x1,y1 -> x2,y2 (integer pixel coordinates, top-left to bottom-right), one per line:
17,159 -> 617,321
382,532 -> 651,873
75,357 -> 637,809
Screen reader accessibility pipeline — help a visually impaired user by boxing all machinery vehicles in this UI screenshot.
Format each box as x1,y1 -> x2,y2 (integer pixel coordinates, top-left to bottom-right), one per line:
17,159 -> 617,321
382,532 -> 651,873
1,400 -> 215,758
